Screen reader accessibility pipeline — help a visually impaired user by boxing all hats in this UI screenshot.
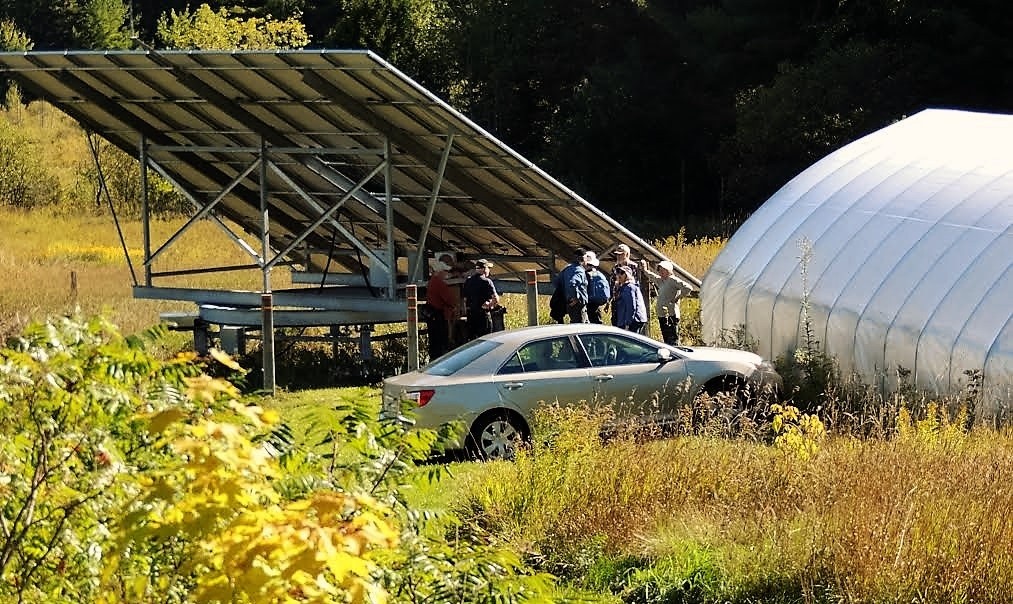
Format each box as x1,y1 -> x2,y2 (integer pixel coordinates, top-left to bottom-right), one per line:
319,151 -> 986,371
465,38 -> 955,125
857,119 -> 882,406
656,260 -> 674,275
581,251 -> 600,266
614,244 -> 631,255
432,261 -> 452,272
476,259 -> 493,269
616,266 -> 633,283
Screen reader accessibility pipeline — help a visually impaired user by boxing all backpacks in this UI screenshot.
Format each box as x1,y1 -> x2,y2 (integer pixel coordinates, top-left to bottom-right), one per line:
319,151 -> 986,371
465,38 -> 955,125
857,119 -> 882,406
586,271 -> 610,305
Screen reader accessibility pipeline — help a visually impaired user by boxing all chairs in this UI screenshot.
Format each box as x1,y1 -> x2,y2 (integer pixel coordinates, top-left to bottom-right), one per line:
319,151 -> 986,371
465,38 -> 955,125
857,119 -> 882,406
536,342 -> 561,369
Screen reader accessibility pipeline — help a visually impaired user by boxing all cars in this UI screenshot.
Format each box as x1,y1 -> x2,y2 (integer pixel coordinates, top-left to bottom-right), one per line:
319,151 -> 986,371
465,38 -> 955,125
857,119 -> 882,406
379,323 -> 784,463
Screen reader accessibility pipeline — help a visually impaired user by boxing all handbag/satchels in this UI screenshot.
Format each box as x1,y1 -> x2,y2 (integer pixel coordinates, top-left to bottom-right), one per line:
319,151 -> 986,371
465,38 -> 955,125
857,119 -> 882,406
419,302 -> 443,326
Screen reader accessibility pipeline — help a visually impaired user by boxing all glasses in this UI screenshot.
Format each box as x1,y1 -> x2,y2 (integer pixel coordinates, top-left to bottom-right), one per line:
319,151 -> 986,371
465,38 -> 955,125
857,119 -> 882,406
656,266 -> 664,271
616,270 -> 625,276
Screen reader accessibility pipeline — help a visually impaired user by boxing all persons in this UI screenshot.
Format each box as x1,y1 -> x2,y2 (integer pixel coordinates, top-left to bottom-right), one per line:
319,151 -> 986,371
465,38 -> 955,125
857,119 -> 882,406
641,260 -> 694,347
550,244 -> 648,334
425,254 -> 500,365
